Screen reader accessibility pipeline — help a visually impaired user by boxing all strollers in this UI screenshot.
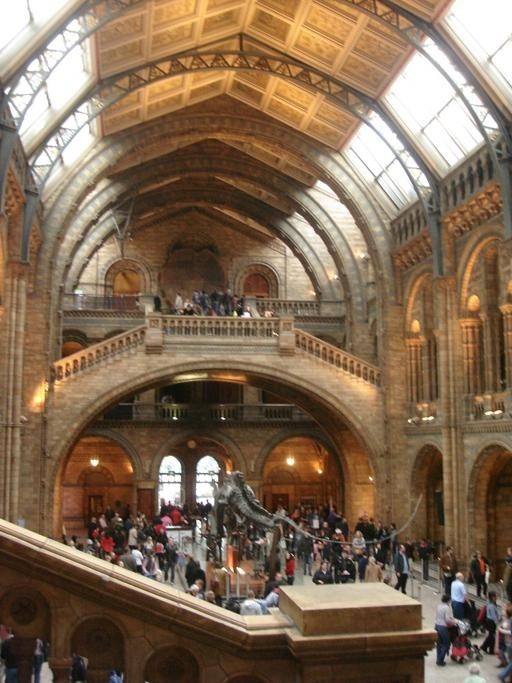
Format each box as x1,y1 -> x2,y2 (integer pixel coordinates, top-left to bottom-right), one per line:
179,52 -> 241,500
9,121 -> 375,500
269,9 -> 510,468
445,618 -> 484,664
333,556 -> 356,583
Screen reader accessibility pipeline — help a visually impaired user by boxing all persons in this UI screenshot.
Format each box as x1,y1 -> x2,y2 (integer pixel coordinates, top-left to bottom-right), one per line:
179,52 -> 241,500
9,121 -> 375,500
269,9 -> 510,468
153,289 -> 275,319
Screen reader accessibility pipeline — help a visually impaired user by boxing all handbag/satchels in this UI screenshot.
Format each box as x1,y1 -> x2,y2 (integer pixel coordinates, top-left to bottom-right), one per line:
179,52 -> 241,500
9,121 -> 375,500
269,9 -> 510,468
484,564 -> 491,584
476,605 -> 486,623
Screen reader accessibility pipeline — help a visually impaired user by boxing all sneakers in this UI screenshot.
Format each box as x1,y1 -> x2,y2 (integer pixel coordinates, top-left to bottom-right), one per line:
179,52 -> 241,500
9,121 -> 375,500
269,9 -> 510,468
451,655 -> 464,663
436,661 -> 446,666
480,642 -> 512,683
